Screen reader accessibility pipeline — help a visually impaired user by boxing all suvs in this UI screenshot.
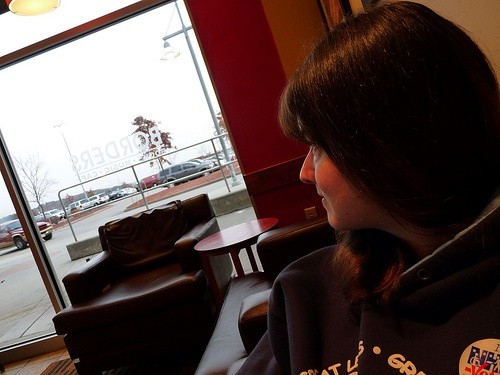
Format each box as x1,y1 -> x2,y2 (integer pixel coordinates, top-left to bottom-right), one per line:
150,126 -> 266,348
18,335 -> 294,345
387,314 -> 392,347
0,219 -> 53,250
41,209 -> 66,220
63,193 -> 110,214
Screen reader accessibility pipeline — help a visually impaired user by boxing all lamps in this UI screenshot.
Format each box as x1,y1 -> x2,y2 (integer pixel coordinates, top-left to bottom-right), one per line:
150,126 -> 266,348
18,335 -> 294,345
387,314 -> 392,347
8,0 -> 61,15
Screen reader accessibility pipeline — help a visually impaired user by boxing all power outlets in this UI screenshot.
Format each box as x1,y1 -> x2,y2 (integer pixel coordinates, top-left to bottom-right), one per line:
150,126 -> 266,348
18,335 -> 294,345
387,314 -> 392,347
305,206 -> 318,219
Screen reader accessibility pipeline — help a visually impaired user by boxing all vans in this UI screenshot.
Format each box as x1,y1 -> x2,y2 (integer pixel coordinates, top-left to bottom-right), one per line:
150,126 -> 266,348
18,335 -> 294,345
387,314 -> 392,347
139,174 -> 161,190
158,162 -> 213,188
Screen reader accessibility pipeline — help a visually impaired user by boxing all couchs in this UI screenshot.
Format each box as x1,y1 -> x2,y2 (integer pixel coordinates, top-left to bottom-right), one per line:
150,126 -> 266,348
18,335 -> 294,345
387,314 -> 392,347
194,211 -> 335,375
52,193 -> 233,375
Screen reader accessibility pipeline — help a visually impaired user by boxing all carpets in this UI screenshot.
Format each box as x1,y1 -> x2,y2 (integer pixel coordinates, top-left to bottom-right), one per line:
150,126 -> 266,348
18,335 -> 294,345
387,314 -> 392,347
40,358 -> 79,375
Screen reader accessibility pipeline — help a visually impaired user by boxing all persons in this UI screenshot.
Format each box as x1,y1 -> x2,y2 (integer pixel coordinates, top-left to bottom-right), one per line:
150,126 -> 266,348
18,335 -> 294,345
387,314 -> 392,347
234,0 -> 500,375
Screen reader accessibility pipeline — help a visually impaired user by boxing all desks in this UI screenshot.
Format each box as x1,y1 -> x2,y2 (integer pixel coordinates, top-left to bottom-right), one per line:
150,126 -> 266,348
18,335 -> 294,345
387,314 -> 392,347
194,218 -> 281,301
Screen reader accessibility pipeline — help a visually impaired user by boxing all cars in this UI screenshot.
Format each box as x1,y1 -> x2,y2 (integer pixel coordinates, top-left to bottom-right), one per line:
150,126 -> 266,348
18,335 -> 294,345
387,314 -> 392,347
110,188 -> 138,199
189,150 -> 235,166
